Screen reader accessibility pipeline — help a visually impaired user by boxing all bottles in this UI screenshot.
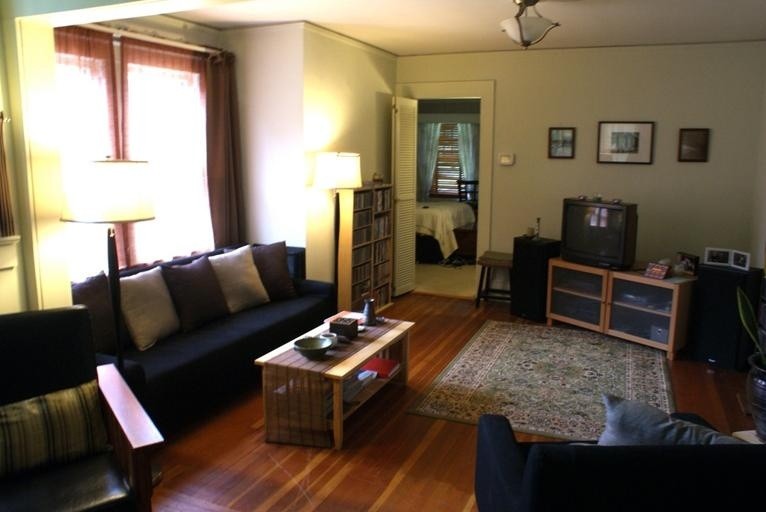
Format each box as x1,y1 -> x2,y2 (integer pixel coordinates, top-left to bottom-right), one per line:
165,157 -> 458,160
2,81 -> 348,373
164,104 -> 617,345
363,298 -> 377,326
536,217 -> 541,241
372,172 -> 384,183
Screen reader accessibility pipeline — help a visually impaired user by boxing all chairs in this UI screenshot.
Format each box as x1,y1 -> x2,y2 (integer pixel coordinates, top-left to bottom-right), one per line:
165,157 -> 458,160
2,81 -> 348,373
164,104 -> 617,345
0,303 -> 166,512
456,179 -> 478,203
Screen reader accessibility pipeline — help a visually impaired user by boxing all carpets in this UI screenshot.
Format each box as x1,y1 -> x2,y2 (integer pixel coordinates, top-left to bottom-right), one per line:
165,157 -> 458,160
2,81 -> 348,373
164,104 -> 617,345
404,317 -> 676,447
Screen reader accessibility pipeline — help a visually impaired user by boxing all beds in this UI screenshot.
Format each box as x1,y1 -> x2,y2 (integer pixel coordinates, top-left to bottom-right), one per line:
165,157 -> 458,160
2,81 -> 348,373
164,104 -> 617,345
416,197 -> 476,266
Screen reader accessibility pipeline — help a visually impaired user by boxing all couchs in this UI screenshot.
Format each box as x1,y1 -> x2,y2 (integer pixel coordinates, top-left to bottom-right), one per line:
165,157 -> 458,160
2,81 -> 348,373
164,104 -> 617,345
475,403 -> 766,511
68,238 -> 337,423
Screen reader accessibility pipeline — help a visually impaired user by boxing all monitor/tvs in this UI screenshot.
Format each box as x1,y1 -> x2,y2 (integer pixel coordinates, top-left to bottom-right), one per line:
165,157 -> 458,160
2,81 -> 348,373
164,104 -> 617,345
558,198 -> 639,271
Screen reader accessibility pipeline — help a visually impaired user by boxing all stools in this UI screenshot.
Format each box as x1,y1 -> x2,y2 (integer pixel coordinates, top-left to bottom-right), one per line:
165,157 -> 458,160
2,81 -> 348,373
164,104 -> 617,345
472,249 -> 515,309
453,221 -> 479,261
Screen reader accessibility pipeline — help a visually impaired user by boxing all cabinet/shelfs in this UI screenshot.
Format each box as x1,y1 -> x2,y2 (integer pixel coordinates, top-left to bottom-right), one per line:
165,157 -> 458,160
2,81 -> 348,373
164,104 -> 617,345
545,258 -> 697,361
335,182 -> 397,316
691,264 -> 764,372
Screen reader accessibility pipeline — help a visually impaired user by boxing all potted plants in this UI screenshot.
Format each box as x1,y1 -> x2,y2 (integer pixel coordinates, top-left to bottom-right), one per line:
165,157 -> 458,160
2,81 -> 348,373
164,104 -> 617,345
729,285 -> 766,440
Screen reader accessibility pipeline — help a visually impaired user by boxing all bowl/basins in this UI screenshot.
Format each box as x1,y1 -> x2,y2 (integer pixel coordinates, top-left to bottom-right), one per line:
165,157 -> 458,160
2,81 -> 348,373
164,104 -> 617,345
292,337 -> 332,359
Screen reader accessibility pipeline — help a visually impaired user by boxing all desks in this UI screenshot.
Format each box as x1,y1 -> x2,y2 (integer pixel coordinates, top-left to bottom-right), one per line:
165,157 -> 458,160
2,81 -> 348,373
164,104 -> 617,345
510,236 -> 561,324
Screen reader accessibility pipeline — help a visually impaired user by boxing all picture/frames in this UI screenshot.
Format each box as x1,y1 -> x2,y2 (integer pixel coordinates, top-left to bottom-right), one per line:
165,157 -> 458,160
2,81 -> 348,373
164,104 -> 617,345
596,119 -> 657,166
729,249 -> 751,272
679,126 -> 712,164
702,246 -> 732,267
547,125 -> 577,160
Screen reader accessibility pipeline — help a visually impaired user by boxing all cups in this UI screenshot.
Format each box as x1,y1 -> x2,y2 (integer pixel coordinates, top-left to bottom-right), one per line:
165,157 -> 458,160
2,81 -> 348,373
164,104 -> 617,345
320,333 -> 338,348
376,317 -> 384,323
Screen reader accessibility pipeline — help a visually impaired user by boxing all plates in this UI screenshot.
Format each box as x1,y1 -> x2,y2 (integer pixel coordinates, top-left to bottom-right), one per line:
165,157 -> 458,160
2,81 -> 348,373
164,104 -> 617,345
357,325 -> 365,332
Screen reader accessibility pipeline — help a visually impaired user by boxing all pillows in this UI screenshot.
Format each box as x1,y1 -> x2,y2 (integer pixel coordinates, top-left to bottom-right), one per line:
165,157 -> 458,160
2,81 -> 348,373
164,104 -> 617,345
116,240 -> 296,355
592,388 -> 742,446
1,377 -> 105,478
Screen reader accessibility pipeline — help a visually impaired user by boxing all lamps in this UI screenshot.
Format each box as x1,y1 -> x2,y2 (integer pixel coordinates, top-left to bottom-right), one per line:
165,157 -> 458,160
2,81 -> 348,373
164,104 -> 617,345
55,154 -> 159,262
313,150 -> 363,307
500,0 -> 561,51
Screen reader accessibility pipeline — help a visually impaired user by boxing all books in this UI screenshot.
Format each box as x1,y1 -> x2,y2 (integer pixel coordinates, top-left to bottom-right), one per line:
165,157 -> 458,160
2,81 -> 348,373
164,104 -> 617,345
272,357 -> 403,419
351,185 -> 393,314
323,308 -> 366,326
